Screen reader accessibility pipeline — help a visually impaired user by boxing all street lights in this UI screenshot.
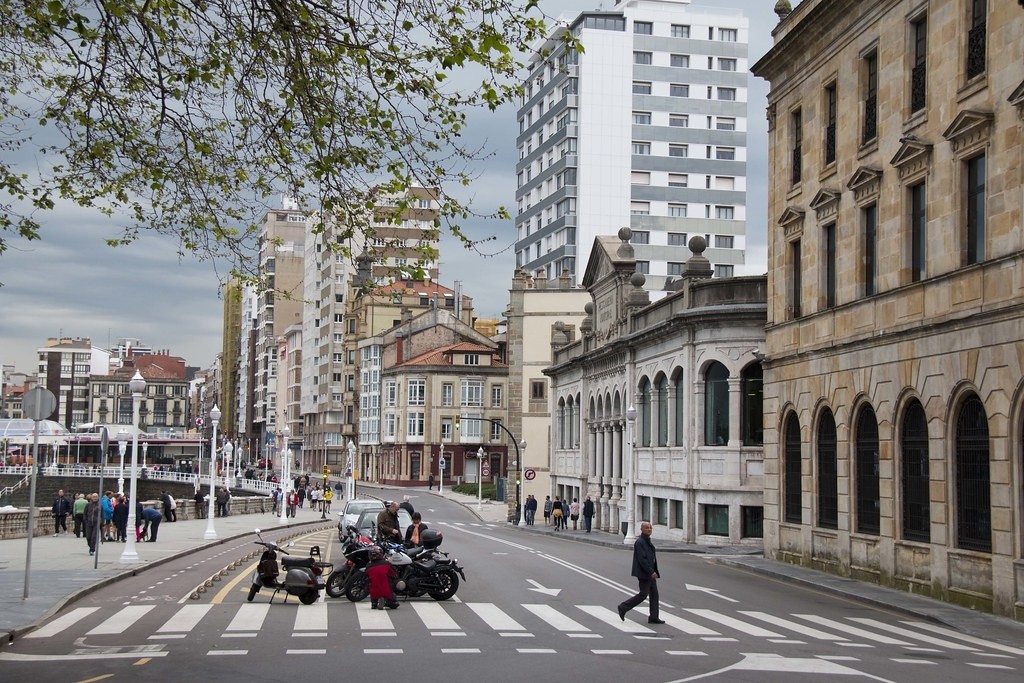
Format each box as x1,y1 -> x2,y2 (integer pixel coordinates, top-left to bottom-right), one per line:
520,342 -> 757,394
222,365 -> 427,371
518,440 -> 528,527
348,440 -> 355,501
117,428 -> 128,497
119,368 -> 147,563
439,443 -> 445,494
623,403 -> 640,546
221,423 -> 305,525
478,446 -> 486,512
204,404 -> 223,540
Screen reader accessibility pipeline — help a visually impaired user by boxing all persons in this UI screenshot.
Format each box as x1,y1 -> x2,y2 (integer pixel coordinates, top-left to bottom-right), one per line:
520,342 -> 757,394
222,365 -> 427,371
194,490 -> 206,519
617,522 -> 665,624
269,488 -> 298,518
235,467 -> 277,489
399,495 -> 414,516
157,489 -> 177,522
57,462 -> 101,475
429,473 -> 433,490
291,474 -> 343,514
217,488 -> 230,517
153,464 -> 199,480
51,489 -> 162,556
0,461 -> 27,473
366,546 -> 400,610
524,494 -> 537,526
294,459 -> 300,470
377,502 -> 402,544
405,512 -> 428,547
543,495 -> 595,533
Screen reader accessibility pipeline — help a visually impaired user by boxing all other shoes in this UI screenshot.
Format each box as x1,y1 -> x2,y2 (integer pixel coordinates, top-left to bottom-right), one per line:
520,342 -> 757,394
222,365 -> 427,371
108,537 -> 116,541
101,540 -> 109,542
648,617 -> 665,623
617,605 -> 626,621
372,605 -> 375,608
65,531 -> 68,534
146,539 -> 156,542
527,522 -> 591,532
377,597 -> 385,610
53,533 -> 58,536
90,551 -> 93,555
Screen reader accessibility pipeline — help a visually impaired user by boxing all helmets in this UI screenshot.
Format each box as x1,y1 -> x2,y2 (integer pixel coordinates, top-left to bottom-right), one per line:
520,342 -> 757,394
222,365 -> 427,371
369,546 -> 384,561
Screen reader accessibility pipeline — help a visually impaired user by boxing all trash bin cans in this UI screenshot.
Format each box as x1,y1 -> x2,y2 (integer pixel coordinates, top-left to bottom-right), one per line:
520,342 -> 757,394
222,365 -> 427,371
622,522 -> 628,536
307,467 -> 312,475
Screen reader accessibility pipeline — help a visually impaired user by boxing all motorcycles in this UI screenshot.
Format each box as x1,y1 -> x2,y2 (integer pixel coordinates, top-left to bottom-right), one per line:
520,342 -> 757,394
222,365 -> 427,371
327,524 -> 472,601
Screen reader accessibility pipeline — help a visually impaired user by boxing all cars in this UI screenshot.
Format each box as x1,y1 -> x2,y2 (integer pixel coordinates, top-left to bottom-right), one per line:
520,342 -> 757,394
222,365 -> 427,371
234,459 -> 247,469
337,500 -> 387,543
225,458 -> 234,467
347,507 -> 415,543
258,458 -> 272,469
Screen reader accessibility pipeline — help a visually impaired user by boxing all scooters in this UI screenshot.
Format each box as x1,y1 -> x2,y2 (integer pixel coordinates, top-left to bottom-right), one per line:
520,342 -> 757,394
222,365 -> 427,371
247,527 -> 334,606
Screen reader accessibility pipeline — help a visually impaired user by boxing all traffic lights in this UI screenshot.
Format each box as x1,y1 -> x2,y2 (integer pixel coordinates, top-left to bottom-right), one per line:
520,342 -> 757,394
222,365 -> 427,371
323,466 -> 327,480
